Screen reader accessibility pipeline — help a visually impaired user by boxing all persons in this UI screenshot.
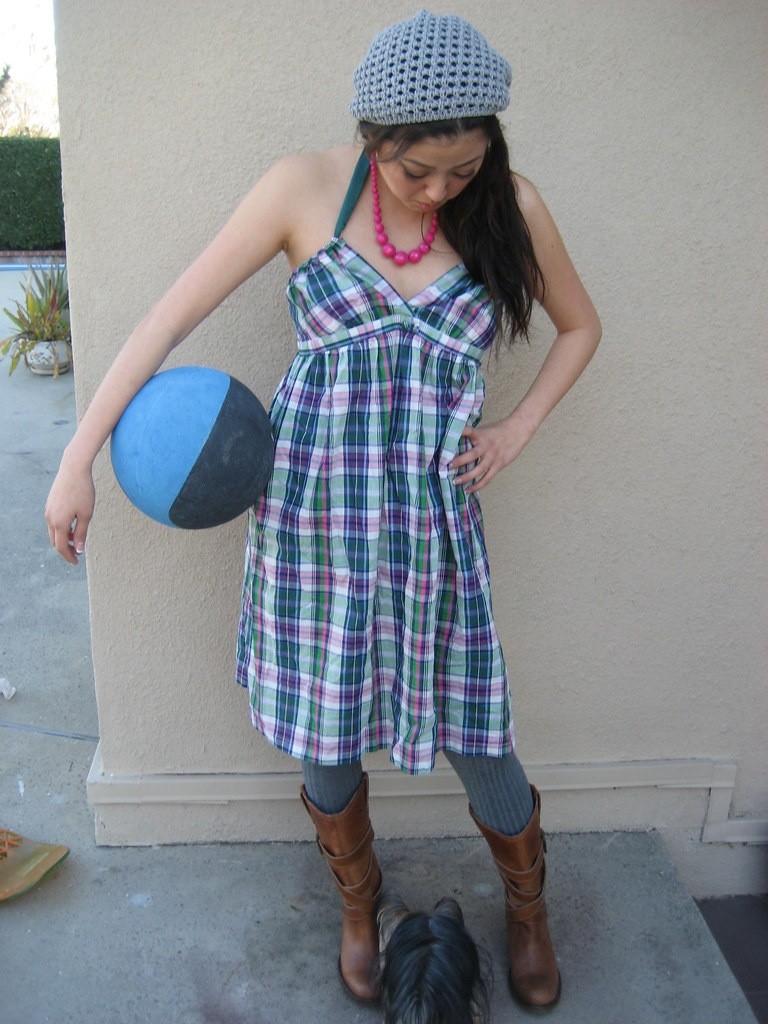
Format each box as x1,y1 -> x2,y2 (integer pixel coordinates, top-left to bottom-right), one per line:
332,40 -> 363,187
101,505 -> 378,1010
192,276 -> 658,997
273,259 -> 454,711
44,13 -> 604,1015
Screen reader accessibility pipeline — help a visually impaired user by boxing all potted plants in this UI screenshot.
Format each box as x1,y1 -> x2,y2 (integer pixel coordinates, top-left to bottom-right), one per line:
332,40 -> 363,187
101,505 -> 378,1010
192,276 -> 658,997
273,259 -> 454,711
0,251 -> 74,379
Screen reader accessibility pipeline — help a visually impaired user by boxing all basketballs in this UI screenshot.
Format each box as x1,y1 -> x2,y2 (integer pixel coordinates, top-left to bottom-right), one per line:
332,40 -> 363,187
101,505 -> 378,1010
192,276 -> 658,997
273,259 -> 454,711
109,366 -> 276,530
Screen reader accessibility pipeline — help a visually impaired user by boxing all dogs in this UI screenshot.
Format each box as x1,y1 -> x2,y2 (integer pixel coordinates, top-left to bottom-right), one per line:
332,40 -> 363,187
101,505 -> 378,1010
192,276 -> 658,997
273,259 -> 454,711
366,897 -> 494,1024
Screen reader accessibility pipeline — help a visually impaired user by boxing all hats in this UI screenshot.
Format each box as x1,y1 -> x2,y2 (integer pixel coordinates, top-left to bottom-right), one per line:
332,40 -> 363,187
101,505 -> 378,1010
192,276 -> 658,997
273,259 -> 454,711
350,10 -> 513,126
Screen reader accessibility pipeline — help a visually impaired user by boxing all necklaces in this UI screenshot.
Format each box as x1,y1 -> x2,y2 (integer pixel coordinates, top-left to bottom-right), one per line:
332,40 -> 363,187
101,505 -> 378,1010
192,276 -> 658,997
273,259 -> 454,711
365,145 -> 442,268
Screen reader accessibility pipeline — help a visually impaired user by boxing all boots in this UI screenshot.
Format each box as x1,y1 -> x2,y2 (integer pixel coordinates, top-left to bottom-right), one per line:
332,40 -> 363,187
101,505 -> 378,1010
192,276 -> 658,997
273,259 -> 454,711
468,783 -> 561,1009
301,772 -> 384,1003
